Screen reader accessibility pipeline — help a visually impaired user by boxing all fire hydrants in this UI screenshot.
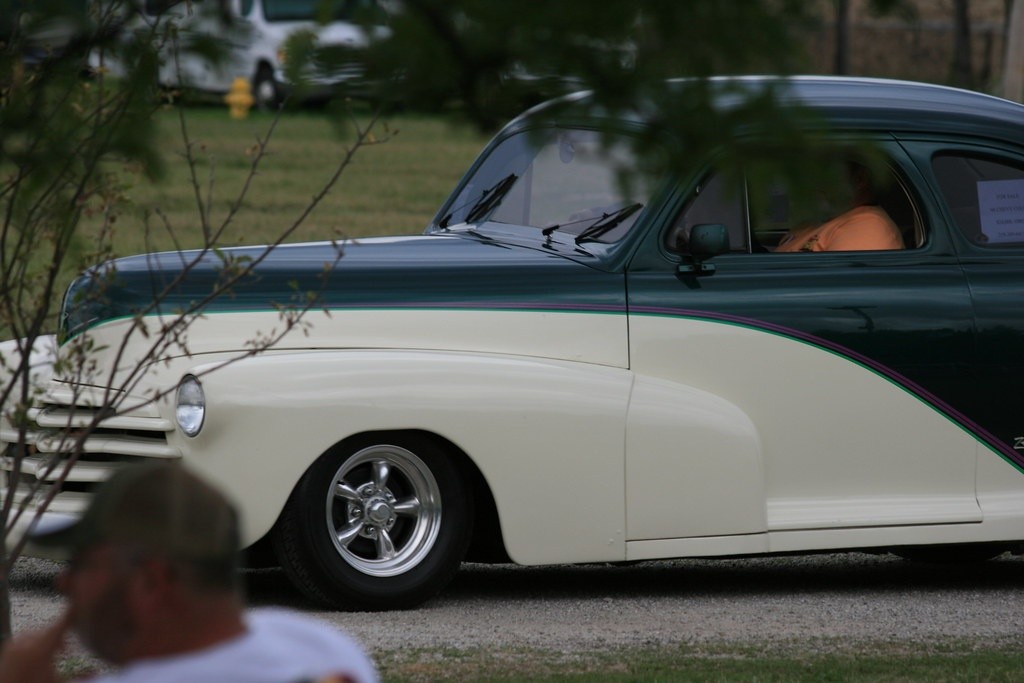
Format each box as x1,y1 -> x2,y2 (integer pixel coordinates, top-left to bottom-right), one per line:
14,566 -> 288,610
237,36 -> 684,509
222,78 -> 256,119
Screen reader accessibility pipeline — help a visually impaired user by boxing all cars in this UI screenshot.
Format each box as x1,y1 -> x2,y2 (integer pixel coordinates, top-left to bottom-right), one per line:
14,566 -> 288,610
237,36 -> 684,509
0,75 -> 1024,609
86,0 -> 398,103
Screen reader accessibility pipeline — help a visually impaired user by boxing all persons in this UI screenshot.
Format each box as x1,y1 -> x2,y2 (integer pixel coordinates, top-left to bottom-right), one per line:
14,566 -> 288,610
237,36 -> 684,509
0,462 -> 382,683
767,149 -> 907,250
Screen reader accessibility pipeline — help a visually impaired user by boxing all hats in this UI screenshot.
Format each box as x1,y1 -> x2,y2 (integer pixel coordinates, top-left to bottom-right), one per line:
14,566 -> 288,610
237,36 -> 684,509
27,462 -> 241,571
